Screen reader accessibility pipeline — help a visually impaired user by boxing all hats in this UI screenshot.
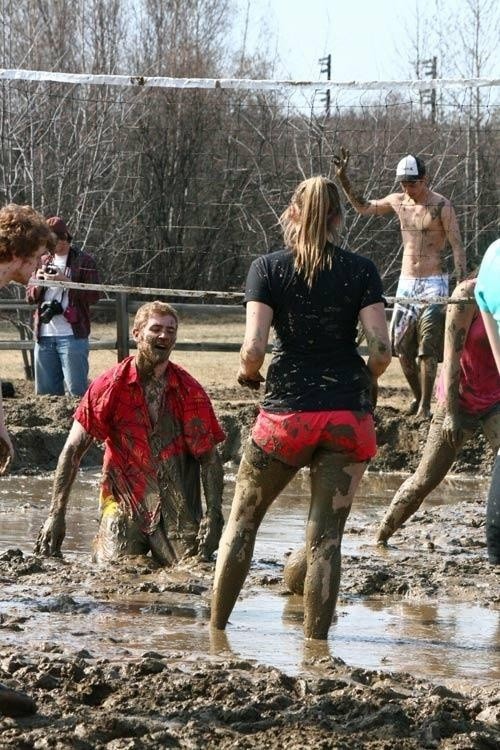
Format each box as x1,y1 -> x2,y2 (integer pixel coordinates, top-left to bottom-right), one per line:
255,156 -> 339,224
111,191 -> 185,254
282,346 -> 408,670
393,152 -> 425,185
45,216 -> 73,241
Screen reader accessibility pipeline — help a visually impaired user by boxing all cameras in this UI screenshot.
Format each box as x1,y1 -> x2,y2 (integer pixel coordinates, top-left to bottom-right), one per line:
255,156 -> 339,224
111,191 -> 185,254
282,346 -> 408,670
39,299 -> 63,323
45,265 -> 56,275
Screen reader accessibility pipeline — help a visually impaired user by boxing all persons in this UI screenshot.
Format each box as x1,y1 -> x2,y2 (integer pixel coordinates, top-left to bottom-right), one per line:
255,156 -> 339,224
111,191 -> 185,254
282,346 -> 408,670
0,201 -> 59,482
472,237 -> 500,566
327,145 -> 469,423
207,172 -> 397,644
32,297 -> 227,569
369,257 -> 500,549
24,215 -> 104,401
